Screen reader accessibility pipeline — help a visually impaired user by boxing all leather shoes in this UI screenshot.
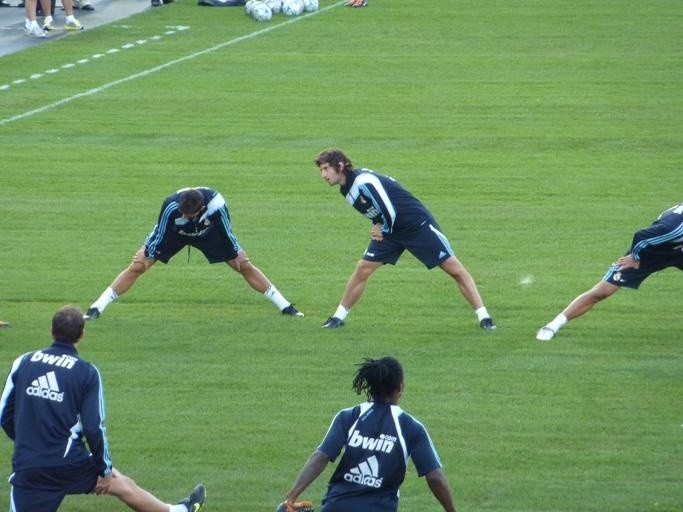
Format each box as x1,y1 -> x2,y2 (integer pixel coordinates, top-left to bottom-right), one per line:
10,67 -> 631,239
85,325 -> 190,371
61,6 -> 78,9
82,5 -> 94,10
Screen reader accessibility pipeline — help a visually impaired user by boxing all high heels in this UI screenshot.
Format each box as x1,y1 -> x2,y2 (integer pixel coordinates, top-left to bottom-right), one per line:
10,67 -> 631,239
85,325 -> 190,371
344,1 -> 368,7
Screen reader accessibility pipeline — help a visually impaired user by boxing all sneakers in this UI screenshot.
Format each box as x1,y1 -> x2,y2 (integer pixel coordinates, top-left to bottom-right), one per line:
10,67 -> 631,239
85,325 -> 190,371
162,0 -> 172,4
0,0 -> 10,6
18,1 -> 25,8
282,305 -> 304,317
536,324 -> 555,341
151,1 -> 160,7
322,317 -> 344,328
83,308 -> 100,321
480,318 -> 496,331
24,10 -> 83,37
179,484 -> 206,512
277,502 -> 312,512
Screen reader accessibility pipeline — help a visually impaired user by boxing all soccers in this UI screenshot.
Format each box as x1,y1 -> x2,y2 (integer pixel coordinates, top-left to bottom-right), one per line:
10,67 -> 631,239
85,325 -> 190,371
244,0 -> 321,21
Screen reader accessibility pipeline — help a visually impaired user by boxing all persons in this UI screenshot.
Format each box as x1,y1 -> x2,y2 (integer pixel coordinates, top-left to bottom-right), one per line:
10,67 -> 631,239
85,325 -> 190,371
83,185 -> 305,320
278,356 -> 457,512
535,201 -> 683,341
0,305 -> 207,512
314,147 -> 496,331
22,0 -> 95,38
